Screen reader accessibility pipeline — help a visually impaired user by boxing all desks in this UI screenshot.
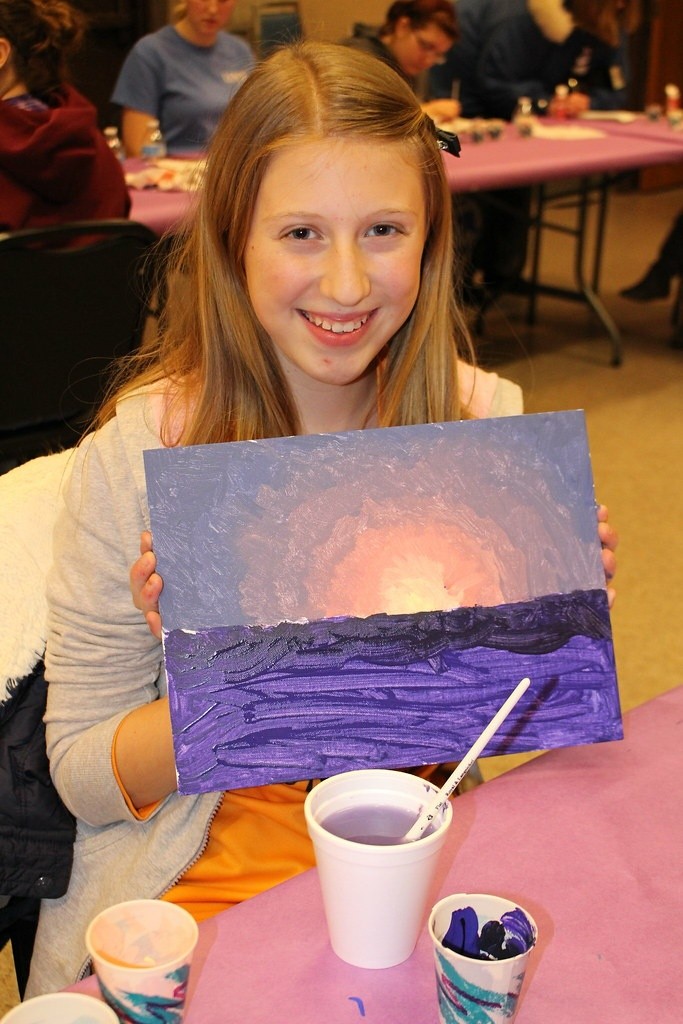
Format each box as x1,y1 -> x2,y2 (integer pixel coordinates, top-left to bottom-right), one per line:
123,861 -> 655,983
10,688 -> 683,1024
121,105 -> 683,366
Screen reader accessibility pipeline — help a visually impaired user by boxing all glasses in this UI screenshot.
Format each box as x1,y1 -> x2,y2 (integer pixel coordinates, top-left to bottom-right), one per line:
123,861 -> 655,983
410,28 -> 447,67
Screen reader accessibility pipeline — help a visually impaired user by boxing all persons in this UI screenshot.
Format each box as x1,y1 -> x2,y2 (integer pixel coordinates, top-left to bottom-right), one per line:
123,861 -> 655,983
427,0 -> 641,290
612,208 -> 683,348
15,38 -> 622,1006
107,1 -> 258,164
347,0 -> 463,127
0,0 -> 133,251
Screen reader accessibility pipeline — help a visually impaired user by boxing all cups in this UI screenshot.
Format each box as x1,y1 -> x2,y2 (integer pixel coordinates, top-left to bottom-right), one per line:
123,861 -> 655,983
86,899 -> 199,1024
0,992 -> 120,1024
428,892 -> 539,1024
302,770 -> 452,969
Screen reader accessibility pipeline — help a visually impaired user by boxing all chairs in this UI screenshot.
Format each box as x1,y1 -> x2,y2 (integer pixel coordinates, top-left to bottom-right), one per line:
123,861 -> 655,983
0,215 -> 167,475
0,447 -> 78,1000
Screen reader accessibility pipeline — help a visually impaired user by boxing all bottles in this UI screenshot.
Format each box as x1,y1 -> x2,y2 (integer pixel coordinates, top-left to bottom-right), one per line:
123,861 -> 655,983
139,119 -> 167,160
104,127 -> 125,163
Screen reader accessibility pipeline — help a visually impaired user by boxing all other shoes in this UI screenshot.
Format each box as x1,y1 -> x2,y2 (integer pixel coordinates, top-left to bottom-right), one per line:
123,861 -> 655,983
621,264 -> 669,301
